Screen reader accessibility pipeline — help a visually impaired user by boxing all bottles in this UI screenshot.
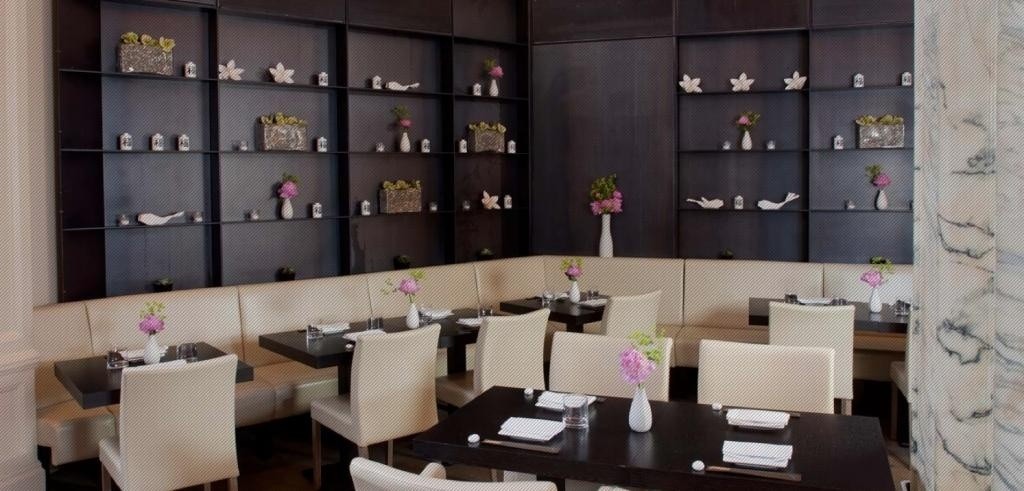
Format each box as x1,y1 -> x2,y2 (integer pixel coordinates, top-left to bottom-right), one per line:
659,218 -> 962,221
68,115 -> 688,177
118,133 -> 132,150
420,137 -> 431,153
507,140 -> 515,154
311,203 -> 323,220
360,200 -> 370,216
371,75 -> 382,90
503,195 -> 512,209
733,196 -> 744,211
853,72 -> 865,88
901,72 -> 913,86
184,61 -> 196,79
177,134 -> 189,151
318,71 -> 327,87
151,134 -> 164,151
458,138 -> 467,153
472,82 -> 481,96
833,134 -> 845,150
316,136 -> 327,153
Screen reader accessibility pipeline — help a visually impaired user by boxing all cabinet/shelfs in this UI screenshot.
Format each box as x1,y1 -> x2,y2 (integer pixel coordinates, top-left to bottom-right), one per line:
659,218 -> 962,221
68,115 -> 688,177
50,0 -> 536,304
668,21 -> 917,263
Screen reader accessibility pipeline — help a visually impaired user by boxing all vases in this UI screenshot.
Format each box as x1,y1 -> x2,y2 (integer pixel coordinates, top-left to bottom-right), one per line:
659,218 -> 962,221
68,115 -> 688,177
486,80 -> 498,99
280,197 -> 294,221
597,212 -> 616,261
397,129 -> 411,154
873,187 -> 888,212
740,130 -> 752,153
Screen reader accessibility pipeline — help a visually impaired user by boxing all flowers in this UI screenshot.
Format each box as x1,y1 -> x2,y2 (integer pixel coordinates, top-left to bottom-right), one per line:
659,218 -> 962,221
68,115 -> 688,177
728,106 -> 763,133
861,159 -> 892,192
585,170 -> 625,222
480,57 -> 506,80
389,99 -> 411,131
271,171 -> 301,200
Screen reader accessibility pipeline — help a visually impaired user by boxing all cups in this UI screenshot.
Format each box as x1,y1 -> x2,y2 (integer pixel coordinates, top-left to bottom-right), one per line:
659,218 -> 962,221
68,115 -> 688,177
784,293 -> 798,304
107,348 -> 130,369
561,394 -> 589,430
367,316 -> 386,331
895,297 -> 910,316
562,430 -> 590,460
543,294 -> 554,307
588,289 -> 600,302
481,308 -> 494,317
176,341 -> 199,365
420,311 -> 434,325
307,322 -> 323,338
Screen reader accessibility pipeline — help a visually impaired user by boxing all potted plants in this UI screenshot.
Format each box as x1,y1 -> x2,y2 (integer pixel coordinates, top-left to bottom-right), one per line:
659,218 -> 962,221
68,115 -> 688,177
111,28 -> 177,80
463,118 -> 508,156
375,176 -> 424,215
851,110 -> 908,154
251,109 -> 311,155
